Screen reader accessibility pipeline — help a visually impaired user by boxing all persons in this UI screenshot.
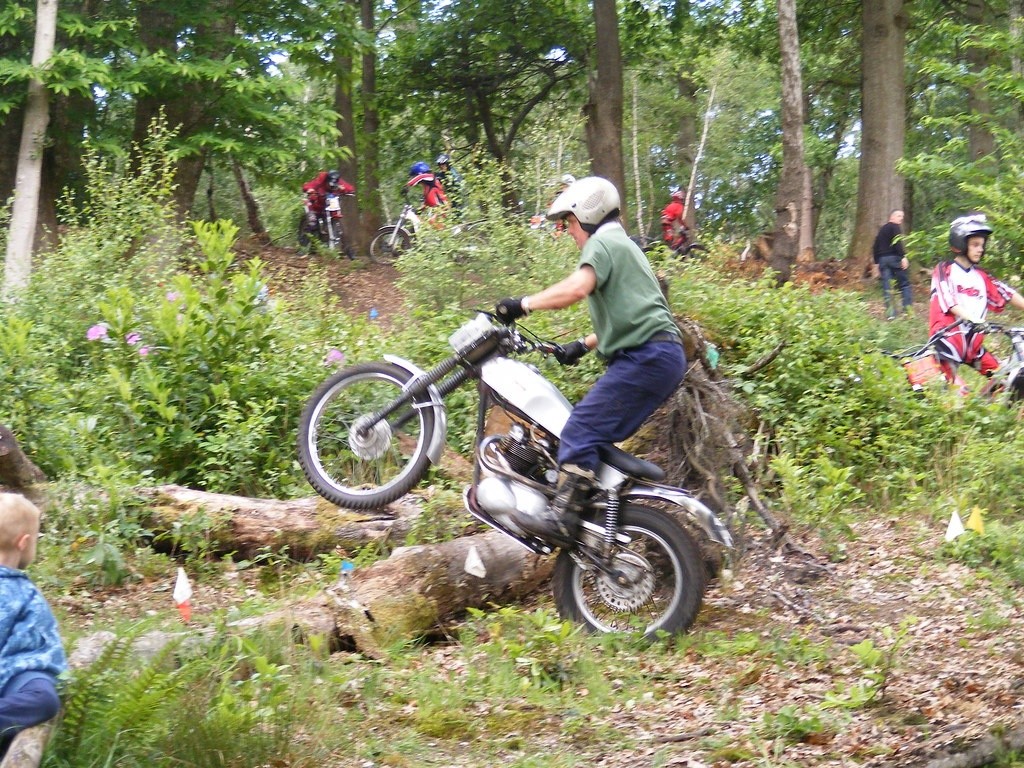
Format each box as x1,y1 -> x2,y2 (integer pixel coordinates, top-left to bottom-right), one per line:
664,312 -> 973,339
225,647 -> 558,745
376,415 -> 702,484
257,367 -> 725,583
495,176 -> 684,549
660,191 -> 690,251
545,174 -> 579,231
302,170 -> 357,220
435,154 -> 467,225
0,492 -> 66,759
398,161 -> 453,234
874,209 -> 915,318
927,215 -> 1024,404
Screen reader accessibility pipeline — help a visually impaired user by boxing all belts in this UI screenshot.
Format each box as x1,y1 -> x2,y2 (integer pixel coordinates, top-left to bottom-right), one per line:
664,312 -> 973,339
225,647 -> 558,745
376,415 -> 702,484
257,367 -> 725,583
650,333 -> 684,345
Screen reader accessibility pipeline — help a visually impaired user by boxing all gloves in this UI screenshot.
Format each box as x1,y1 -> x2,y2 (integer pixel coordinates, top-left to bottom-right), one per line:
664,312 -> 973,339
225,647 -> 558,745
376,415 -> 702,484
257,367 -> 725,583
964,319 -> 991,335
495,295 -> 533,324
552,338 -> 591,366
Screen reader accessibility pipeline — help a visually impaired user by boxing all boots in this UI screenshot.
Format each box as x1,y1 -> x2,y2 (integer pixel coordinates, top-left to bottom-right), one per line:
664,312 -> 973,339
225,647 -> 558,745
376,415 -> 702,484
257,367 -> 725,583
509,467 -> 599,550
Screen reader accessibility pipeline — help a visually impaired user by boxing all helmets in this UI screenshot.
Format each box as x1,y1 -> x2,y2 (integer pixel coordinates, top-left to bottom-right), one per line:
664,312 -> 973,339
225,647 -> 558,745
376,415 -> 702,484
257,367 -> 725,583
948,213 -> 993,254
407,161 -> 432,175
671,190 -> 685,200
435,154 -> 450,163
328,169 -> 339,183
544,177 -> 621,232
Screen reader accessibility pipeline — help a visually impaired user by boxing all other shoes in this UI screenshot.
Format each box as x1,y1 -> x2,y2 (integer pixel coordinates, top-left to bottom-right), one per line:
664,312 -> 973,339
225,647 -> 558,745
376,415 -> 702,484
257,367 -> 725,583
348,252 -> 358,260
297,240 -> 311,259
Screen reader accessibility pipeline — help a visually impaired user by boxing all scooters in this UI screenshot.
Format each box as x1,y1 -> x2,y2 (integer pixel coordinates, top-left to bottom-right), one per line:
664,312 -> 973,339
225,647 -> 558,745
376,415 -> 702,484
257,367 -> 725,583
630,225 -> 710,263
837,315 -> 1024,420
509,191 -> 569,276
298,192 -> 356,261
296,305 -> 735,649
367,188 -> 490,271
